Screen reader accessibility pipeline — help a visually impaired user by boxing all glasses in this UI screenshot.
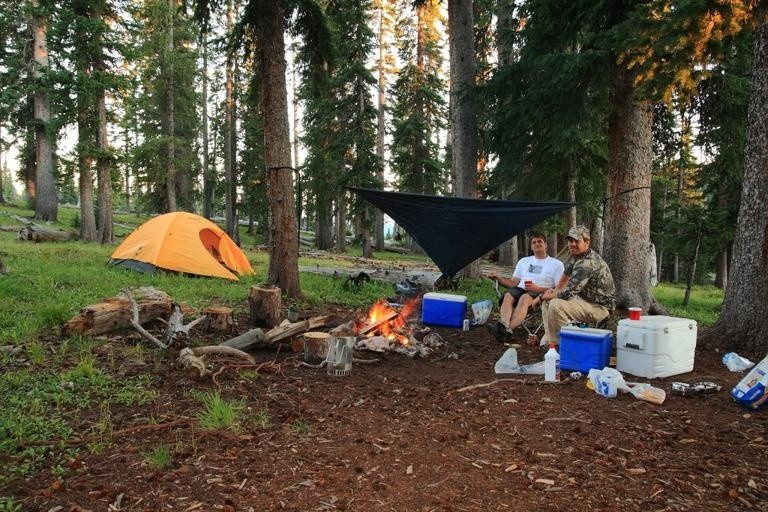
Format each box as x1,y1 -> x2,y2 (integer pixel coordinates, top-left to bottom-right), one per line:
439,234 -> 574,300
566,321 -> 588,328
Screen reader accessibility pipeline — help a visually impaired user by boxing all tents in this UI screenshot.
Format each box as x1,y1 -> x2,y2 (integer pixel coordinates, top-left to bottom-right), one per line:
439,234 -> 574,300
107,209 -> 258,286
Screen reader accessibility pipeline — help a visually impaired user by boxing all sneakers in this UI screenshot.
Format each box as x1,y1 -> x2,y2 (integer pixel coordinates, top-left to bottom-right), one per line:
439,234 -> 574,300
496,320 -> 512,336
488,323 -> 503,341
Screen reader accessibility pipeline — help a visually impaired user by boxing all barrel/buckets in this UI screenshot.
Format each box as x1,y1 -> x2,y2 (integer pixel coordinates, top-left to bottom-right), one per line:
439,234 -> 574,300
324,334 -> 354,377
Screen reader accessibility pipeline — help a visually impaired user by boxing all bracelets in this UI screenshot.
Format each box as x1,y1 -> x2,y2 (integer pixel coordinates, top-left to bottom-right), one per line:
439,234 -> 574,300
538,293 -> 544,301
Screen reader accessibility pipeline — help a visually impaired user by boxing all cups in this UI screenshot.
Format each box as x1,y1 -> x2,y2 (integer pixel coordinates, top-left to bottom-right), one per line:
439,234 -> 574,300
524,280 -> 532,289
628,307 -> 641,320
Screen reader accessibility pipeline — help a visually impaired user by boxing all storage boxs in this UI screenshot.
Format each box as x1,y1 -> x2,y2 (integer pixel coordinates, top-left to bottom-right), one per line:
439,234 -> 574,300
422,292 -> 468,328
558,314 -> 698,379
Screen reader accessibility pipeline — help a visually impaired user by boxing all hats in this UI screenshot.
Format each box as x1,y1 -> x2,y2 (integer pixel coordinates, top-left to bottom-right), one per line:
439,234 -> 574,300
565,225 -> 591,241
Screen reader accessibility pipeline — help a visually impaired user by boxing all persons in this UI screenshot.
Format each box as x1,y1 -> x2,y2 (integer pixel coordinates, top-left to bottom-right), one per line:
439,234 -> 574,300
531,224 -> 620,349
484,231 -> 568,343
